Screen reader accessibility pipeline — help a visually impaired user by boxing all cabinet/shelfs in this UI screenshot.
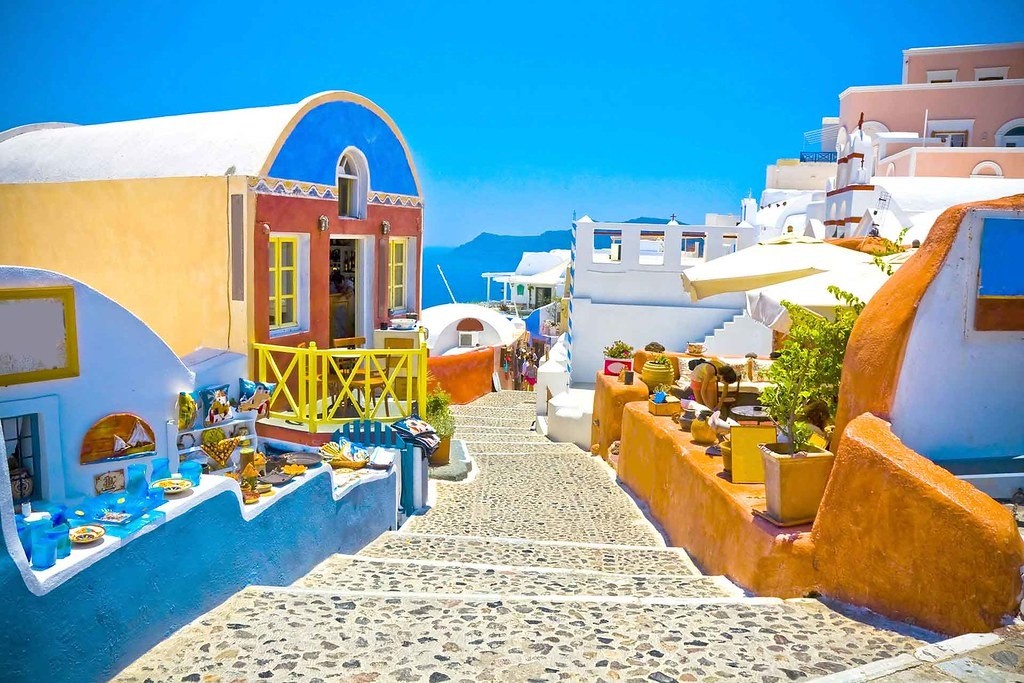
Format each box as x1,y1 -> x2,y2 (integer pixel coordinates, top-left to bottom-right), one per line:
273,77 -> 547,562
167,406 -> 258,475
330,246 -> 355,273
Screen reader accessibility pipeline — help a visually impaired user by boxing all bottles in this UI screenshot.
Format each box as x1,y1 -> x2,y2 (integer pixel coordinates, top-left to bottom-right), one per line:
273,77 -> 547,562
344,251 -> 355,272
127,463 -> 148,494
679,409 -> 697,431
151,458 -> 172,483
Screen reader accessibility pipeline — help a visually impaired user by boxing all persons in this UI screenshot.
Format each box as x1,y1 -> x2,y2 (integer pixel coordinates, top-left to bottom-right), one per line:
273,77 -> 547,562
519,347 -> 538,391
690,360 -> 736,412
330,260 -> 355,338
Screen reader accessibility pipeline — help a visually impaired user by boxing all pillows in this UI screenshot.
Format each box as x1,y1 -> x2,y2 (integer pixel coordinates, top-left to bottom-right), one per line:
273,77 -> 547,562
199,384 -> 234,428
177,390 -> 201,433
752,357 -> 776,381
677,357 -> 712,381
717,357 -> 752,383
235,378 -> 277,419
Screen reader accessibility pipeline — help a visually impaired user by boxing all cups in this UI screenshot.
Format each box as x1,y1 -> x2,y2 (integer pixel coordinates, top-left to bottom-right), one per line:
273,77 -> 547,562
148,487 -> 165,501
687,342 -> 707,354
380,318 -> 389,331
178,461 -> 203,486
625,371 -> 634,386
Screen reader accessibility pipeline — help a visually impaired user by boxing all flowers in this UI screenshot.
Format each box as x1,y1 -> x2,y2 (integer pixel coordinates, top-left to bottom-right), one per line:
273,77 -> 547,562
603,340 -> 633,359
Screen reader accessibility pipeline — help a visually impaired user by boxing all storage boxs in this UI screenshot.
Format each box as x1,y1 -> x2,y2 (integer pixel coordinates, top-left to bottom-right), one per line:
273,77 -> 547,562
649,399 -> 681,416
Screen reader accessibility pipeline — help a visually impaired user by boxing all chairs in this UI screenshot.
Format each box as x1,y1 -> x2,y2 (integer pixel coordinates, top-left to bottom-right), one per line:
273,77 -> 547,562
384,337 -> 414,380
297,337 -> 389,417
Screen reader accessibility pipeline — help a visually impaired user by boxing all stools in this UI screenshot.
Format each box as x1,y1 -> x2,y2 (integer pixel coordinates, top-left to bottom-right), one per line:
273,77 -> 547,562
718,395 -> 736,420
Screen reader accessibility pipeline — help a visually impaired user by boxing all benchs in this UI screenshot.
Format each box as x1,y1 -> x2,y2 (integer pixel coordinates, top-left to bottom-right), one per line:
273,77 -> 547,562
671,381 -> 779,406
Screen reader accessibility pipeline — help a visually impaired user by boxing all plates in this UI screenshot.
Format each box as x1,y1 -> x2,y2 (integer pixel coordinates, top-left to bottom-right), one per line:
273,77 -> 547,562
149,478 -> 192,494
279,452 -> 324,465
255,470 -> 293,484
69,525 -> 106,544
685,351 -> 704,356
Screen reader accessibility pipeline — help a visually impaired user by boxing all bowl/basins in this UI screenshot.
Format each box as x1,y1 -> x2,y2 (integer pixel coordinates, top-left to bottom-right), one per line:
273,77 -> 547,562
390,319 -> 419,329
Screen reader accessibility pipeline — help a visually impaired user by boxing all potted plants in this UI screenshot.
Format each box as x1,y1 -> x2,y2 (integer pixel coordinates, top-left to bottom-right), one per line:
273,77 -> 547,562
758,286 -> 866,523
426,408 -> 456,466
641,353 -> 674,392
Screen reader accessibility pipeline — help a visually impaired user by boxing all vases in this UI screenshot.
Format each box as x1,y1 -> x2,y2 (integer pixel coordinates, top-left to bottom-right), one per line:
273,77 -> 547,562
691,410 -> 717,443
719,440 -> 732,472
126,464 -> 148,494
680,409 -> 697,432
10,468 -> 33,500
151,458 -> 170,482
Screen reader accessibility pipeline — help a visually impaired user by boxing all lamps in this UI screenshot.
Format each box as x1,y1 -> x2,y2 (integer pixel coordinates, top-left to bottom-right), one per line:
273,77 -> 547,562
320,215 -> 329,232
382,220 -> 391,235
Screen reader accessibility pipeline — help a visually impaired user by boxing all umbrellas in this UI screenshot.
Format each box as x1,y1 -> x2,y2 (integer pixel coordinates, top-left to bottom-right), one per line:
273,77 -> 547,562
681,227 -> 920,339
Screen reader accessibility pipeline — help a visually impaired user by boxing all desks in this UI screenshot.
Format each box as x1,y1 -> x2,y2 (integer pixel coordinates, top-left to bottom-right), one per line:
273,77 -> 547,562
328,348 -> 366,416
732,406 -> 790,425
506,305 -> 522,311
